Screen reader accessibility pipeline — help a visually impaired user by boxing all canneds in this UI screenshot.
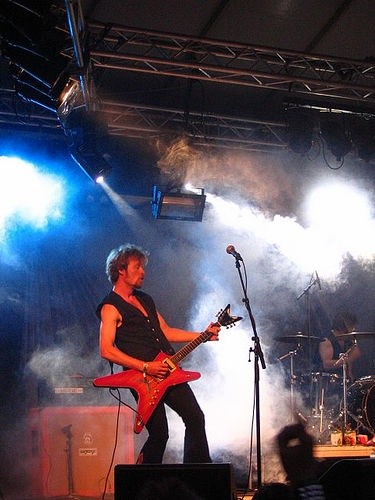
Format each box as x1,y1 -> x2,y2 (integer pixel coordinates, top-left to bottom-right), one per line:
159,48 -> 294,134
343,431 -> 357,446
356,434 -> 367,445
331,431 -> 343,448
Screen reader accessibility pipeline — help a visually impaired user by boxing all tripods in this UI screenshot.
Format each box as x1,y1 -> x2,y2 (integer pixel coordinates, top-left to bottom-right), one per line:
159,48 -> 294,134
316,335 -> 375,445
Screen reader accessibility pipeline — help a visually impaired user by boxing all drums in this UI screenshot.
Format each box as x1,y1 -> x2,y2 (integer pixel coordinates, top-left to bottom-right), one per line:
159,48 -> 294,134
342,378 -> 375,437
297,373 -> 344,420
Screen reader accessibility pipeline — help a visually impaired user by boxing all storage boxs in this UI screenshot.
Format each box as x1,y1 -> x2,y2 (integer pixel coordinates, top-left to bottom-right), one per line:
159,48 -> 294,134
30,406 -> 136,499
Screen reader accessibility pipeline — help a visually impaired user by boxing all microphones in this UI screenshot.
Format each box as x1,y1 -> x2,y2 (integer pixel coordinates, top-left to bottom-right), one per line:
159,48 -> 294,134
61,424 -> 72,433
226,245 -> 243,261
315,270 -> 321,290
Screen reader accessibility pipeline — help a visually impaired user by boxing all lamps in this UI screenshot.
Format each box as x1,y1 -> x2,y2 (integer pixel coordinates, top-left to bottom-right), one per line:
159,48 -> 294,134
150,185 -> 206,222
8,52 -> 80,117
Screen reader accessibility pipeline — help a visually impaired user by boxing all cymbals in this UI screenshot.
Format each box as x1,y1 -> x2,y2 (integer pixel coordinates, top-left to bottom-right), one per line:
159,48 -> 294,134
274,335 -> 326,344
335,331 -> 374,338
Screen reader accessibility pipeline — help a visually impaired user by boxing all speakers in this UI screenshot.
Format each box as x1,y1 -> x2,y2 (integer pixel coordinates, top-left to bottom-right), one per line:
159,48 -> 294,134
26,405 -> 135,500
114,463 -> 237,500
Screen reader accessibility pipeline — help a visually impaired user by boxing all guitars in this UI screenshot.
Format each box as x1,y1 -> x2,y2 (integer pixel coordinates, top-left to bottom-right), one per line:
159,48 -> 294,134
92,303 -> 243,434
